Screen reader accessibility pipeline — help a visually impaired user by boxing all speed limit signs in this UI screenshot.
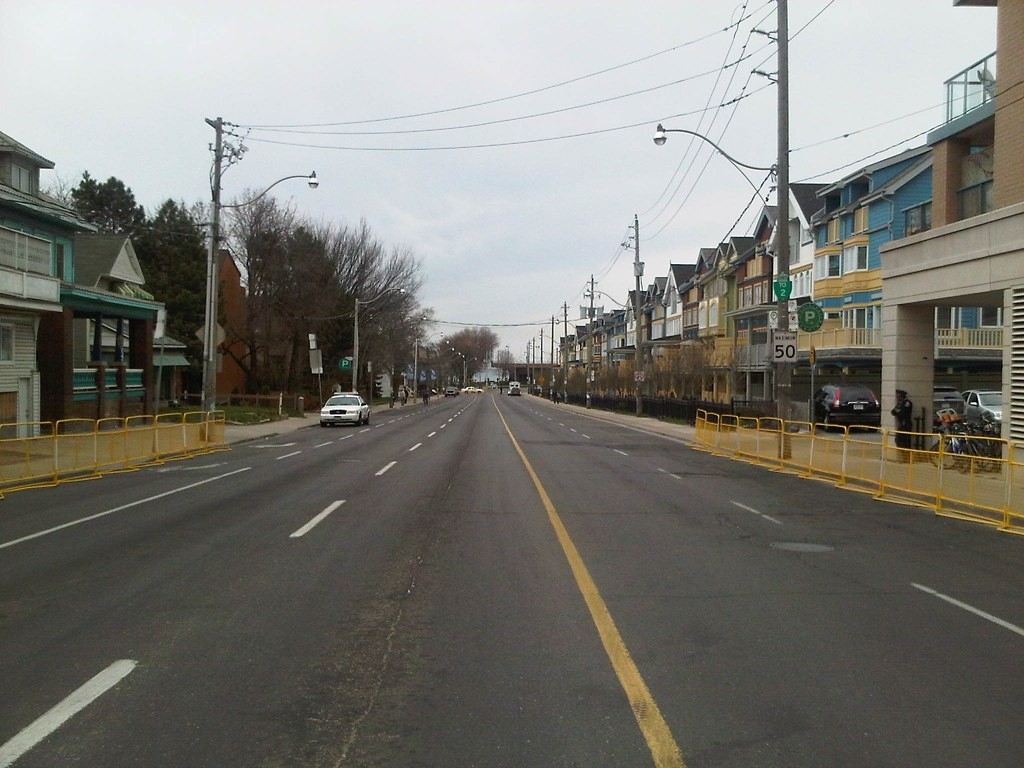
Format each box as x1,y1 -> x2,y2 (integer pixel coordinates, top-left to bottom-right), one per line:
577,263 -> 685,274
772,330 -> 797,362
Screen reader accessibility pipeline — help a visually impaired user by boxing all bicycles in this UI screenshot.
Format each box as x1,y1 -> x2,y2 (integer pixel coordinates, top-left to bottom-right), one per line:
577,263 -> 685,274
928,414 -> 1002,474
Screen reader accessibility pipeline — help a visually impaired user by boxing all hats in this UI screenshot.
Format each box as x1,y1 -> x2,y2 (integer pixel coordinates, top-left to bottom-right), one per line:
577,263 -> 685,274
896,390 -> 908,396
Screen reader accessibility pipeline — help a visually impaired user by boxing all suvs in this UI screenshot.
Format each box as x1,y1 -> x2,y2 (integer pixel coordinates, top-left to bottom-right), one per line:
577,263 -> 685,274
932,385 -> 967,427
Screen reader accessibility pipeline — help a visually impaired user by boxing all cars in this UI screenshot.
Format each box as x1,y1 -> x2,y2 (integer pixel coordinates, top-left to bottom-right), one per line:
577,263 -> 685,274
508,381 -> 521,396
319,391 -> 370,427
445,386 -> 456,398
461,387 -> 484,394
961,390 -> 1002,433
813,383 -> 881,433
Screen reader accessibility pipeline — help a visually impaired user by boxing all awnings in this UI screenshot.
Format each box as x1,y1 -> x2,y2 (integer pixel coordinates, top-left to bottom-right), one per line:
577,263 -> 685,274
152,355 -> 190,366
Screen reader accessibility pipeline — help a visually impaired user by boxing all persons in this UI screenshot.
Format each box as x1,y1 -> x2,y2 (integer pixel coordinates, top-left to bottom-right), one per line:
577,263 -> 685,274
423,389 -> 429,405
552,391 -> 562,404
332,383 -> 346,394
891,390 -> 913,449
400,388 -> 412,406
392,390 -> 396,405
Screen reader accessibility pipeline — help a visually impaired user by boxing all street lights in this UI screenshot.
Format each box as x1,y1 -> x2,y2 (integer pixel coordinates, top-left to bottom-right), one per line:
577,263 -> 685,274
352,286 -> 407,391
651,123 -> 794,461
413,332 -> 444,403
199,170 -> 321,442
